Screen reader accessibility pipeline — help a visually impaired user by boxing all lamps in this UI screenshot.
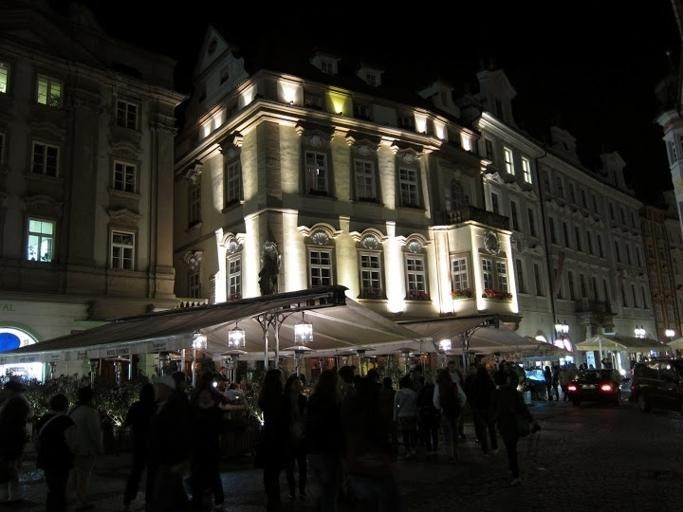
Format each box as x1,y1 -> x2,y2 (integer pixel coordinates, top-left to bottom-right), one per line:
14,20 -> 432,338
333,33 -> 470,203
227,320 -> 246,348
293,312 -> 313,344
192,328 -> 207,352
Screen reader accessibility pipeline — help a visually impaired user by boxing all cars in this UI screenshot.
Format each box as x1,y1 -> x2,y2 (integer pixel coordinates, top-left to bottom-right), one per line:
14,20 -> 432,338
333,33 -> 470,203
566,369 -> 630,407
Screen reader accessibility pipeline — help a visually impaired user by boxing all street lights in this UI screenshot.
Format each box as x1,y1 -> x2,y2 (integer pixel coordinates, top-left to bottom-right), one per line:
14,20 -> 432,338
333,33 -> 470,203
664,328 -> 674,342
553,319 -> 569,352
634,324 -> 645,338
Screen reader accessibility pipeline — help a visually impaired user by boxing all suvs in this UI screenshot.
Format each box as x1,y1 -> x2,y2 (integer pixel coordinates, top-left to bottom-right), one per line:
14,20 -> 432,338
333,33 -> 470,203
629,357 -> 682,415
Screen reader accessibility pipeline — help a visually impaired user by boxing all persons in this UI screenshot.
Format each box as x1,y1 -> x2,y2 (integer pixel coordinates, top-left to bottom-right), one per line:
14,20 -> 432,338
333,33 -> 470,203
0,351 -> 683,510
